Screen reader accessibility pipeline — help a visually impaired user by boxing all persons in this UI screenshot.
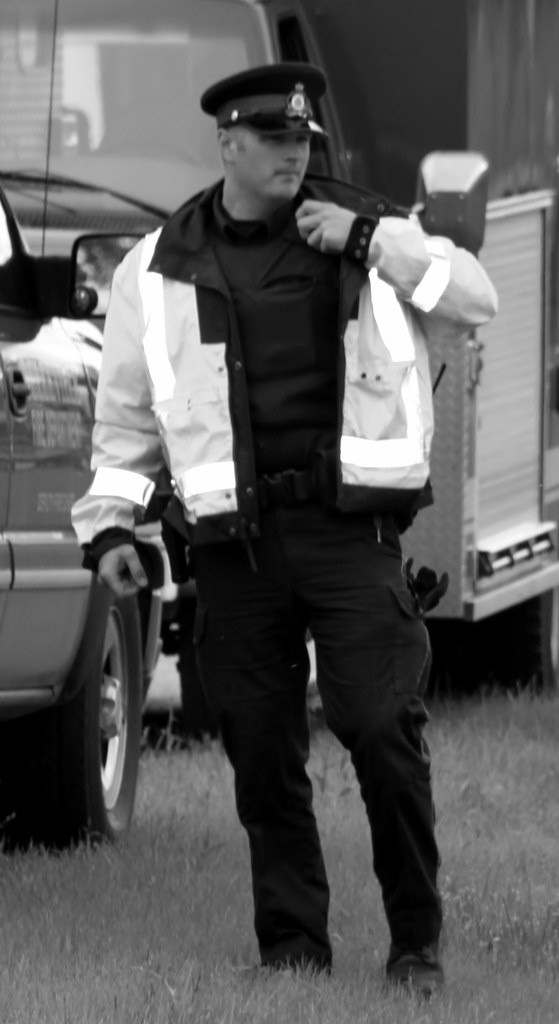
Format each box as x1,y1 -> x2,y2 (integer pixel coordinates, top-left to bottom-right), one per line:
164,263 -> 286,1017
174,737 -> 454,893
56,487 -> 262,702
71,61 -> 496,995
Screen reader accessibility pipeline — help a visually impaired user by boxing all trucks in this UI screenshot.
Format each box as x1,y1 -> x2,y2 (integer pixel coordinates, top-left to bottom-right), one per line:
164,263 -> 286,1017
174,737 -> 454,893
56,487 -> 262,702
1,3 -> 559,690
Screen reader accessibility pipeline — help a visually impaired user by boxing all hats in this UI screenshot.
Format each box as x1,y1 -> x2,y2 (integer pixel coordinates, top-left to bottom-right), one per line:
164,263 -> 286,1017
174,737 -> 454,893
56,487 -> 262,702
201,60 -> 331,142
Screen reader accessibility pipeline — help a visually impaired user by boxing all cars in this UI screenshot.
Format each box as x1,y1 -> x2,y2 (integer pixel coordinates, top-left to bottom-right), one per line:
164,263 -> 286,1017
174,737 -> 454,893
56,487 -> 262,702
0,175 -> 169,843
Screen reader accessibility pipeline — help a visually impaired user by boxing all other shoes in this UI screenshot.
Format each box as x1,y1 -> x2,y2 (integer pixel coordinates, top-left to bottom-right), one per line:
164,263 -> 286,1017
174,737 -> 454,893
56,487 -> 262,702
385,941 -> 446,1002
